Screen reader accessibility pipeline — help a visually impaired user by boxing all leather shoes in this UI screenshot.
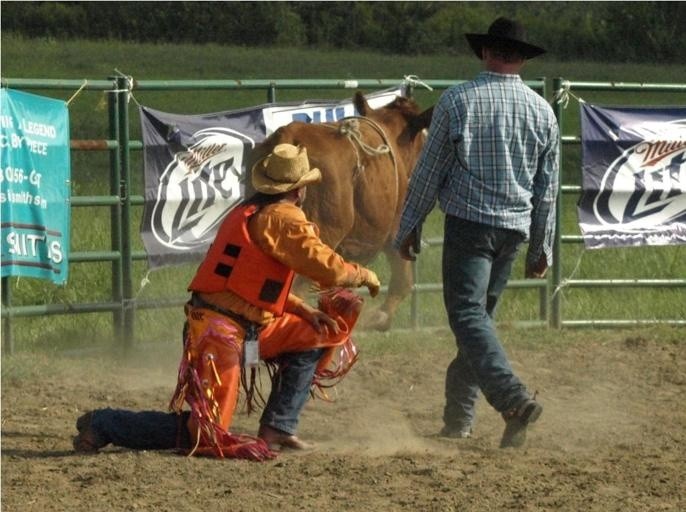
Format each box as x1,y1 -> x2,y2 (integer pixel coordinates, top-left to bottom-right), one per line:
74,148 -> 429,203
498,392 -> 544,448
72,409 -> 102,455
257,424 -> 314,451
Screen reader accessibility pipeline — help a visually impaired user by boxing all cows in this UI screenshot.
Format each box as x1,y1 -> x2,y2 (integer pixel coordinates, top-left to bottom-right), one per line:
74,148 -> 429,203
241,88 -> 436,335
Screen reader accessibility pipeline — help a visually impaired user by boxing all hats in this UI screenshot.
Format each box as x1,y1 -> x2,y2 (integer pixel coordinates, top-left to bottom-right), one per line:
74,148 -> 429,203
464,16 -> 547,60
251,143 -> 323,195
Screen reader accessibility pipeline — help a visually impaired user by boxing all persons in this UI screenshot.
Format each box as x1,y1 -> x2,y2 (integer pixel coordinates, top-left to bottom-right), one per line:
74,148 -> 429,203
394,17 -> 560,450
73,143 -> 381,461
